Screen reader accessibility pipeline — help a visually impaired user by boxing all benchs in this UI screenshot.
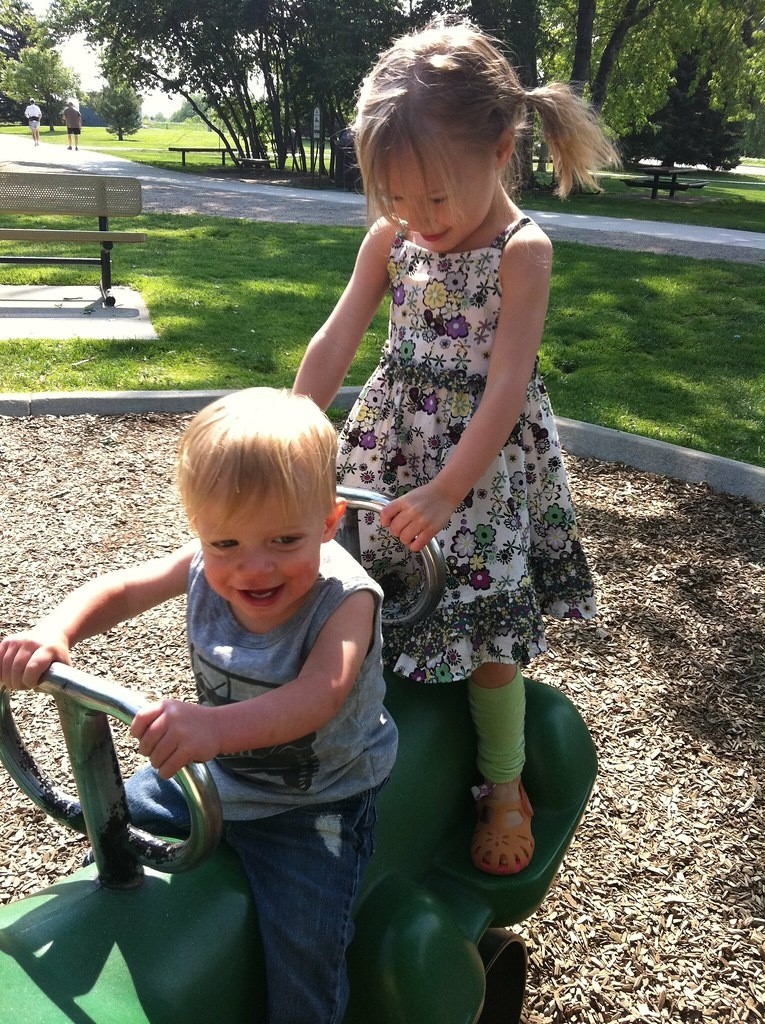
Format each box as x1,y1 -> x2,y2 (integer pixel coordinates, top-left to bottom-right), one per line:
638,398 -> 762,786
233,157 -> 275,179
169,147 -> 237,167
619,178 -> 710,190
0,171 -> 147,306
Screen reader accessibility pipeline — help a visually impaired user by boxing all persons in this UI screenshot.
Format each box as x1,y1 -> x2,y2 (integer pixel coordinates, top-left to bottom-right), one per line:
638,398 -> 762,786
288,27 -> 620,873
25,98 -> 42,147
0,387 -> 399,1024
64,101 -> 81,151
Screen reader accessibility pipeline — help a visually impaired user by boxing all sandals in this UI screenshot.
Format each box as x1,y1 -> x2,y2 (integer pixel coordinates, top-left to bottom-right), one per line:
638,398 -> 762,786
472,781 -> 535,873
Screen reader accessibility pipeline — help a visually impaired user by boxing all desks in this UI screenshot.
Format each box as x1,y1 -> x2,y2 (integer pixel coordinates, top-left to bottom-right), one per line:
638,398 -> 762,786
637,166 -> 698,199
